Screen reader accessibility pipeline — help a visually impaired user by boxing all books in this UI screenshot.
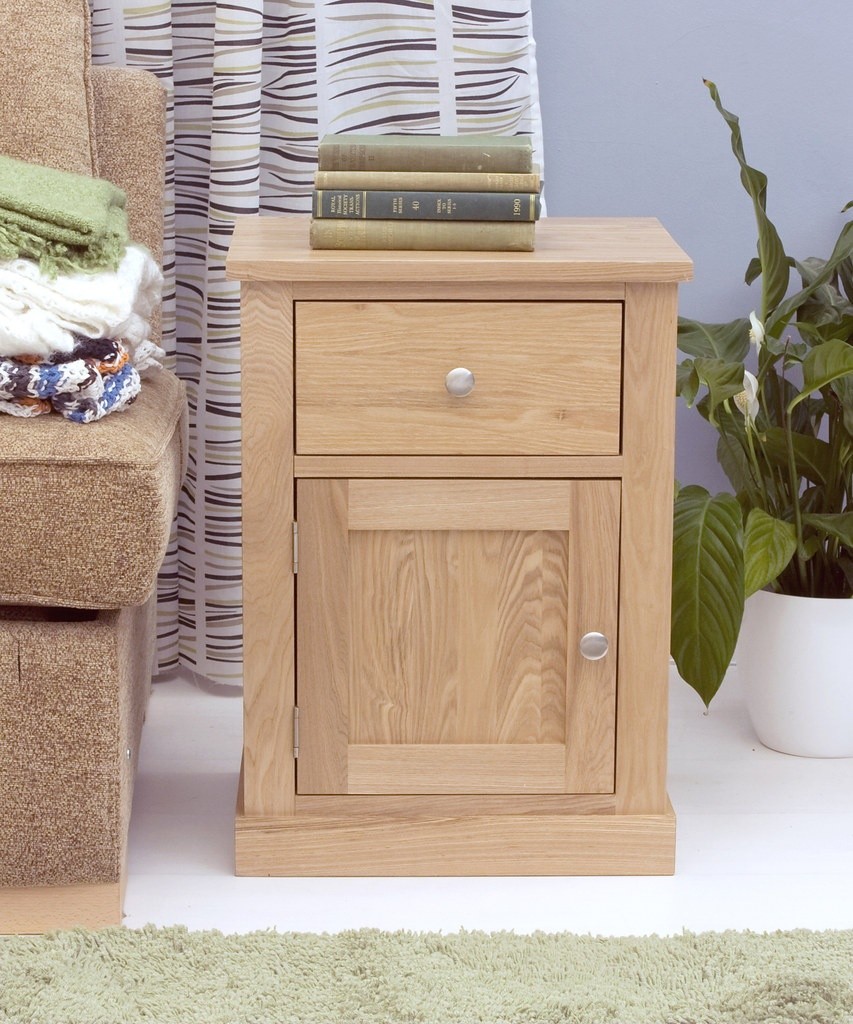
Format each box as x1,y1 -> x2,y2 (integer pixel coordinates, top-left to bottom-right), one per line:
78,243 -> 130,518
309,134 -> 541,253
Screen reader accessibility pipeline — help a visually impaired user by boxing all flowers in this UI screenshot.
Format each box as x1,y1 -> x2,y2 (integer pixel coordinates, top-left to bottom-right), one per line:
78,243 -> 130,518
672,78 -> 853,705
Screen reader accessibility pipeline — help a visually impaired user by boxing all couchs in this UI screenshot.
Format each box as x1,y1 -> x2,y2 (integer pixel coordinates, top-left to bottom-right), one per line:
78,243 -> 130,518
0,0 -> 190,886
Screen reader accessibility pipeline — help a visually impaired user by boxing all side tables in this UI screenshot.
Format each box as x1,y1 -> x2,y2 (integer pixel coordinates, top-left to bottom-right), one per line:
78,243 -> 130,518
225,213 -> 695,875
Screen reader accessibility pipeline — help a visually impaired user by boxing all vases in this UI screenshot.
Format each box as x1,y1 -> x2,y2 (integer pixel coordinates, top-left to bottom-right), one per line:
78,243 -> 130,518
736,583 -> 853,758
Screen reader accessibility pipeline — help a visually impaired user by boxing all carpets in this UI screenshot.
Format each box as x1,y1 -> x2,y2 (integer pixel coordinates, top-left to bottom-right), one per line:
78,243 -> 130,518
0,920 -> 853,1024
